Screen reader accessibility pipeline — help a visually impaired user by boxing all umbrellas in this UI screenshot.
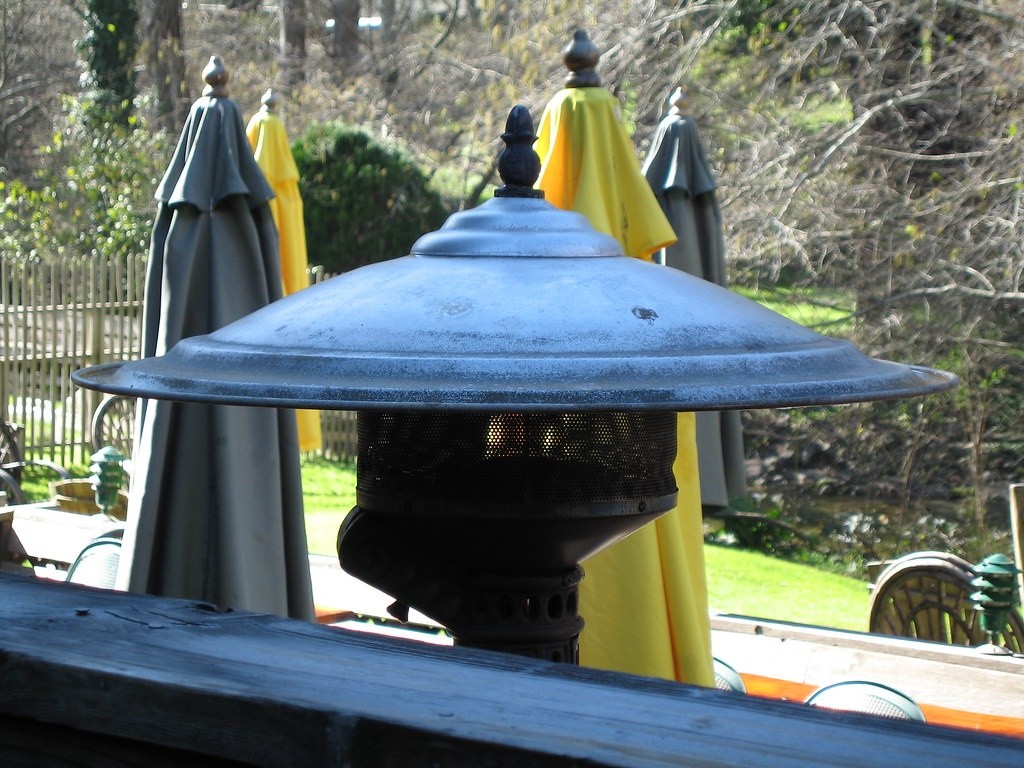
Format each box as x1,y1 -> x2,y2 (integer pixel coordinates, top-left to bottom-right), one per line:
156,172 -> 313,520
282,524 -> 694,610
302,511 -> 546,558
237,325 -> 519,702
117,51 -> 317,623
534,30 -> 762,691
